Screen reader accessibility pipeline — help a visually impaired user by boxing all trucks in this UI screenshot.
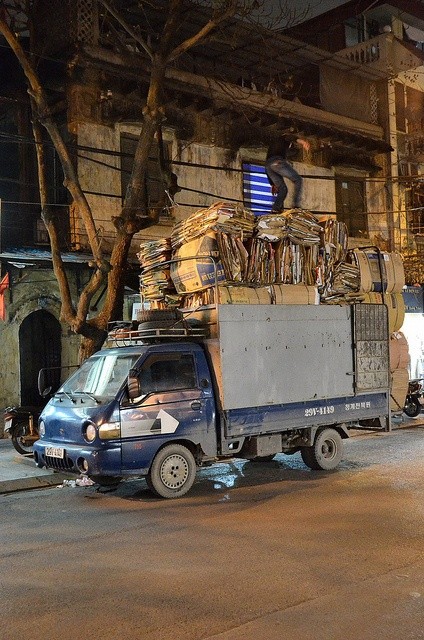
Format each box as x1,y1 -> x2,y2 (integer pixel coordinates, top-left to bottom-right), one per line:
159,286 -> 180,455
31,255 -> 390,498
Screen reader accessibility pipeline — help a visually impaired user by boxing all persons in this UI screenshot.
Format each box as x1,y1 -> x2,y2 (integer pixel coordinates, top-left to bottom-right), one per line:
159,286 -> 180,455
265,131 -> 312,214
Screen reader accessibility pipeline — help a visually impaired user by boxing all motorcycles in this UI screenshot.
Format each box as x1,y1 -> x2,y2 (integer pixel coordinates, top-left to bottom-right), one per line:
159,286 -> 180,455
404,378 -> 424,418
4,405 -> 44,458
